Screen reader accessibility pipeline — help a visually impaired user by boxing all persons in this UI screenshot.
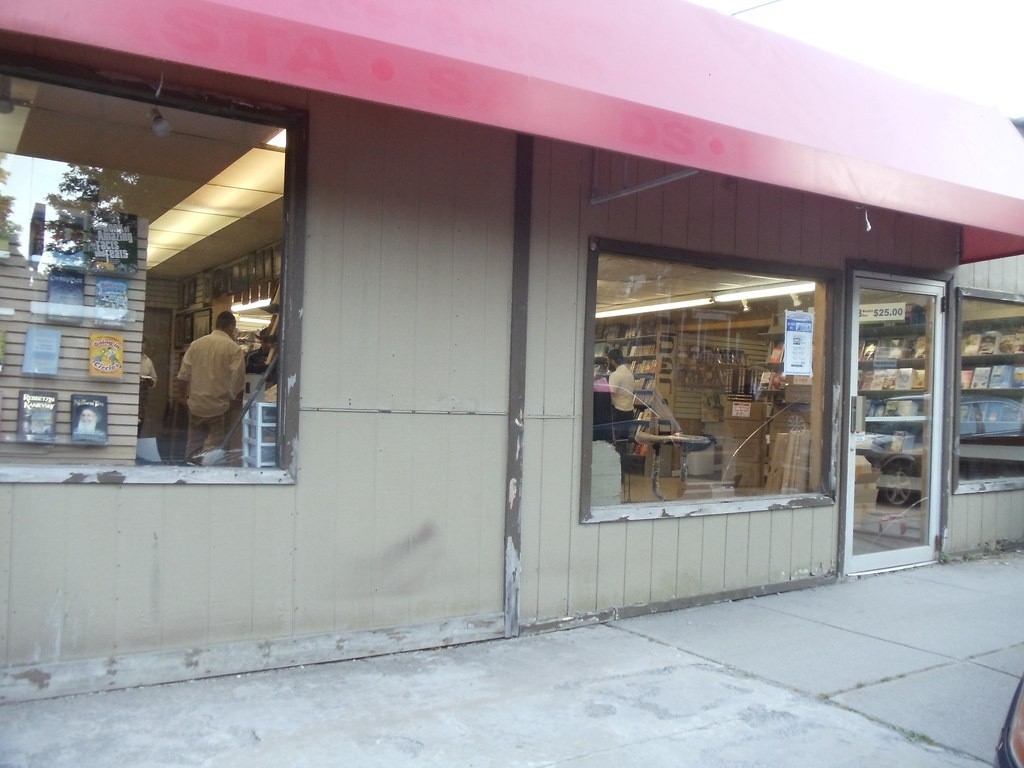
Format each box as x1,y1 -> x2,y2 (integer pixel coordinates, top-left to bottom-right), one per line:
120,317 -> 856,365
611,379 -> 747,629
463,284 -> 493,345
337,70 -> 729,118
607,348 -> 635,486
634,383 -> 686,481
137,334 -> 158,438
594,356 -> 612,447
987,407 -> 999,422
244,326 -> 278,392
176,311 -> 246,466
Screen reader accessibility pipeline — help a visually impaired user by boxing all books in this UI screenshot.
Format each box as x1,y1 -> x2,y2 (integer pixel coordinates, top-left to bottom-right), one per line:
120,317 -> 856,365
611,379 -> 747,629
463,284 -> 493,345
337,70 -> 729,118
634,407 -> 651,420
594,318 -> 675,338
962,331 -> 1024,355
24,326 -> 124,381
858,368 -> 923,390
45,270 -> 129,329
29,209 -> 138,277
890,431 -> 916,454
960,365 -> 1024,389
633,392 -> 654,405
594,341 -> 656,357
635,378 -> 655,389
859,336 -> 925,360
757,370 -> 785,390
866,399 -> 918,417
628,423 -> 648,456
765,340 -> 784,362
15,391 -> 108,446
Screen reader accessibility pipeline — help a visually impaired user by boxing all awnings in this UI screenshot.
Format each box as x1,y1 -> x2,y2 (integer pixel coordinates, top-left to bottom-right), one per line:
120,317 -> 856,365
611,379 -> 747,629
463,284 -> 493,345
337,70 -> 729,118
0,0 -> 1024,266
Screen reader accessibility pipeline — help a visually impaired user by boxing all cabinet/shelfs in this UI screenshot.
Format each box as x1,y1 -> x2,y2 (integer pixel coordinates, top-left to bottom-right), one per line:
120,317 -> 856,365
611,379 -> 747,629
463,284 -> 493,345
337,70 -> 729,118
856,351 -> 1024,491
592,335 -> 657,474
241,400 -> 278,469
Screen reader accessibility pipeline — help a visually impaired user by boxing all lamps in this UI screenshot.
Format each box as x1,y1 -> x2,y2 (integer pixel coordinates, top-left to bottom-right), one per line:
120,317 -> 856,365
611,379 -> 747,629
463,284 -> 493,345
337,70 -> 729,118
864,209 -> 872,231
152,68 -> 172,137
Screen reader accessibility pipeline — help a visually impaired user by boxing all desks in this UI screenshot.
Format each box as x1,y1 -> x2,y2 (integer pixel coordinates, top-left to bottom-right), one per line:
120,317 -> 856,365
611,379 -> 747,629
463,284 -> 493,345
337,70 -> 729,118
639,432 -> 714,499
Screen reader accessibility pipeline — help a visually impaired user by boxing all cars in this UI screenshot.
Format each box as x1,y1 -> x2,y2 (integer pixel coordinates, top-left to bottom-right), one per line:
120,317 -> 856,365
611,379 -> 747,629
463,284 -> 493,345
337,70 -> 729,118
586,375 -> 679,473
866,392 -> 1024,509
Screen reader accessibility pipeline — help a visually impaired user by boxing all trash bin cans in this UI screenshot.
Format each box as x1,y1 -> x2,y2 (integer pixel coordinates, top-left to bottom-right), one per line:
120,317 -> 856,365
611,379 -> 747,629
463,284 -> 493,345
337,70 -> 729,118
687,433 -> 716,476
